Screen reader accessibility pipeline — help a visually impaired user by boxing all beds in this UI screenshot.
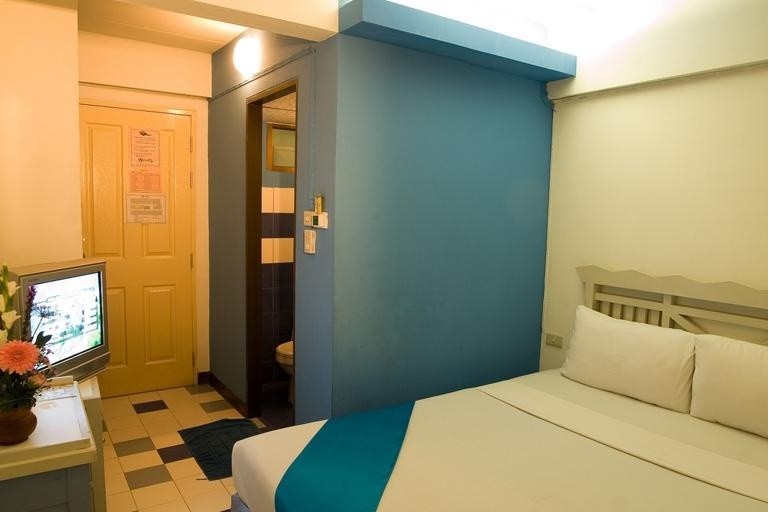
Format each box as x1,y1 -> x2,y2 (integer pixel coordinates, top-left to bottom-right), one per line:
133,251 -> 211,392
224,264 -> 768,511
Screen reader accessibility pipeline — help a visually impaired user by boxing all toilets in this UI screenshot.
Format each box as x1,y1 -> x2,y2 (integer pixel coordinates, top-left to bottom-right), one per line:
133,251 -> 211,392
276,341 -> 294,404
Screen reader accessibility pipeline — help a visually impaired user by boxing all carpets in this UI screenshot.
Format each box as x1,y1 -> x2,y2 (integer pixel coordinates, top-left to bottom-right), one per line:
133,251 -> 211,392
177,418 -> 263,481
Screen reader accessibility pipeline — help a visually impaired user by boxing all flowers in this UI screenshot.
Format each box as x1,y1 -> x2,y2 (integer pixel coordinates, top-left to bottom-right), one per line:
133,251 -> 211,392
0,264 -> 57,404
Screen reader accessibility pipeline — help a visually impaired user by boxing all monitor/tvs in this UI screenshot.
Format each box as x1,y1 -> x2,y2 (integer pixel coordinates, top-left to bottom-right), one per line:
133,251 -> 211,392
7,257 -> 110,384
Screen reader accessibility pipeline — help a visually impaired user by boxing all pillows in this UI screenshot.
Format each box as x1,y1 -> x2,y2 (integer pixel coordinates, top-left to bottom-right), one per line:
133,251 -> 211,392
558,303 -> 768,442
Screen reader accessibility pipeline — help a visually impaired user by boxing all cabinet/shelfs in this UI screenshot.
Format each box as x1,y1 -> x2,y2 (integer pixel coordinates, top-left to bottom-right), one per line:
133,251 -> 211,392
0,376 -> 106,512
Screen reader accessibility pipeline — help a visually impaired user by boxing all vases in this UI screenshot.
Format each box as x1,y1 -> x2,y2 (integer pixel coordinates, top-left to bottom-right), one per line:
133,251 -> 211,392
0,400 -> 39,447
